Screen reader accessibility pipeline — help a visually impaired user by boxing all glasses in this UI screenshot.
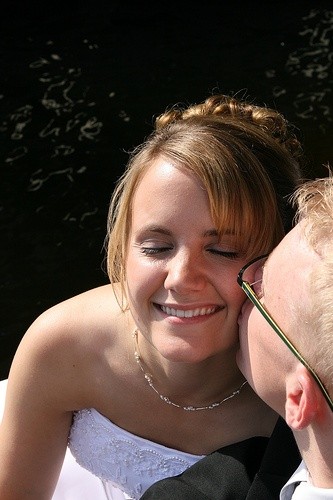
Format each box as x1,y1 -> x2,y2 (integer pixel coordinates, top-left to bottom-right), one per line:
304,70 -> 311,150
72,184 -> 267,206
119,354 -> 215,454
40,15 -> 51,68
236,253 -> 333,414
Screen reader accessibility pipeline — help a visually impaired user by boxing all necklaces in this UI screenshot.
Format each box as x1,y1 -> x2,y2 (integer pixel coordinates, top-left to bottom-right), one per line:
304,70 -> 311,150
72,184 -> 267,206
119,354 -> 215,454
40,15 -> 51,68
135,327 -> 249,413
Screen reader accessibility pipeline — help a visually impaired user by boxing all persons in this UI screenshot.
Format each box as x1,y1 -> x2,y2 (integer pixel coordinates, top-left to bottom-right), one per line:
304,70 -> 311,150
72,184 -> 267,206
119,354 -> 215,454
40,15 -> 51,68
0,100 -> 298,500
129,174 -> 333,500
